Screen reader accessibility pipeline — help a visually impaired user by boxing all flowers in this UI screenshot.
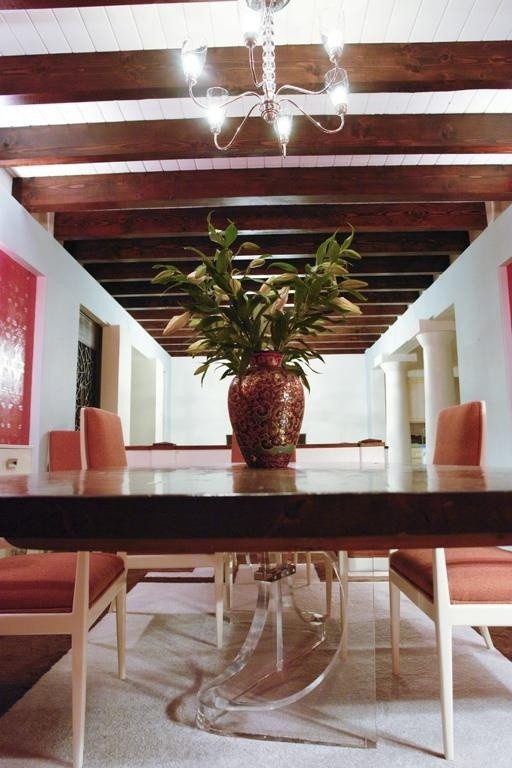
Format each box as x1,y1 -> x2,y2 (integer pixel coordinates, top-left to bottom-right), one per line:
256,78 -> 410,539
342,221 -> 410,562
151,211 -> 374,397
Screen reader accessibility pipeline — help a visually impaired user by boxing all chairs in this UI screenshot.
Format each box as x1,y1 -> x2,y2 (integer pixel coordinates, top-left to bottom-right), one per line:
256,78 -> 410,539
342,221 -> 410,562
79,405 -> 197,574
0,549 -> 127,768
326,402 -> 485,659
388,546 -> 511,759
47,429 -> 80,469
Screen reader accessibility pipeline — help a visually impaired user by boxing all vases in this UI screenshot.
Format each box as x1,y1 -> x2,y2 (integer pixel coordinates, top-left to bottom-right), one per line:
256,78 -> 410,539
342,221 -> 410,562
225,350 -> 309,477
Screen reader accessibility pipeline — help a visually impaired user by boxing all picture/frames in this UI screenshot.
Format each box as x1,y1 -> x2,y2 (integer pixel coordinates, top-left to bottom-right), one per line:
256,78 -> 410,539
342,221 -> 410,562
0,242 -> 46,458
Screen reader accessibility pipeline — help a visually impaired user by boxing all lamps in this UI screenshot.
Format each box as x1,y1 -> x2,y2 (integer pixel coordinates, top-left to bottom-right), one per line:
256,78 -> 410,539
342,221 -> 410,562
179,1 -> 355,157
